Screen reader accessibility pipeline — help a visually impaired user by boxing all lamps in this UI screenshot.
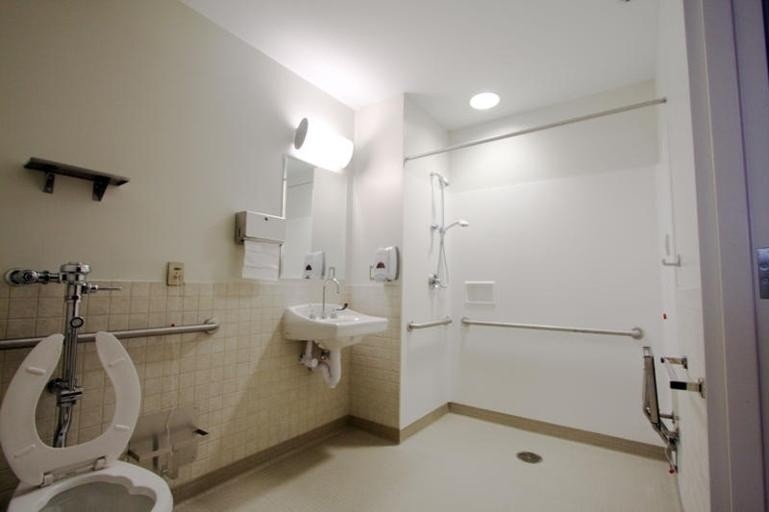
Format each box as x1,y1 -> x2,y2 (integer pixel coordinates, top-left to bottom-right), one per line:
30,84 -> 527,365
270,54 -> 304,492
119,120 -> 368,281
294,118 -> 355,174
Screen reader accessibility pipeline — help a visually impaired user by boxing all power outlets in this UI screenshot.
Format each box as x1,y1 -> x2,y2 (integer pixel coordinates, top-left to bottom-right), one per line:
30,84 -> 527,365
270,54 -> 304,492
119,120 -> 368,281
167,261 -> 185,286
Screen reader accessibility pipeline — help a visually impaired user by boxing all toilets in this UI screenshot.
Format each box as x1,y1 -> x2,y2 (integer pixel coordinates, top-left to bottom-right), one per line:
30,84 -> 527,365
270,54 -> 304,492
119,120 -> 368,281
0,330 -> 174,512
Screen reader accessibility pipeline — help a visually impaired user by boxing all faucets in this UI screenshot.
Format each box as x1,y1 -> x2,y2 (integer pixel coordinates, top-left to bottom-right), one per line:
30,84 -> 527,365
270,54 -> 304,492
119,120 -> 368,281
322,276 -> 342,317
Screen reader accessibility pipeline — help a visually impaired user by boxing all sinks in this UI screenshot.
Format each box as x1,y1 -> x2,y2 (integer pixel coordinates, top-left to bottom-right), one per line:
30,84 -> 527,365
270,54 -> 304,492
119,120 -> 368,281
283,302 -> 387,352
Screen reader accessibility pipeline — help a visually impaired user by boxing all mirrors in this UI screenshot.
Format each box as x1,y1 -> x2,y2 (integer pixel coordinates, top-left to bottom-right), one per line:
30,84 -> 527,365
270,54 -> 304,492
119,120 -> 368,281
282,155 -> 347,280
